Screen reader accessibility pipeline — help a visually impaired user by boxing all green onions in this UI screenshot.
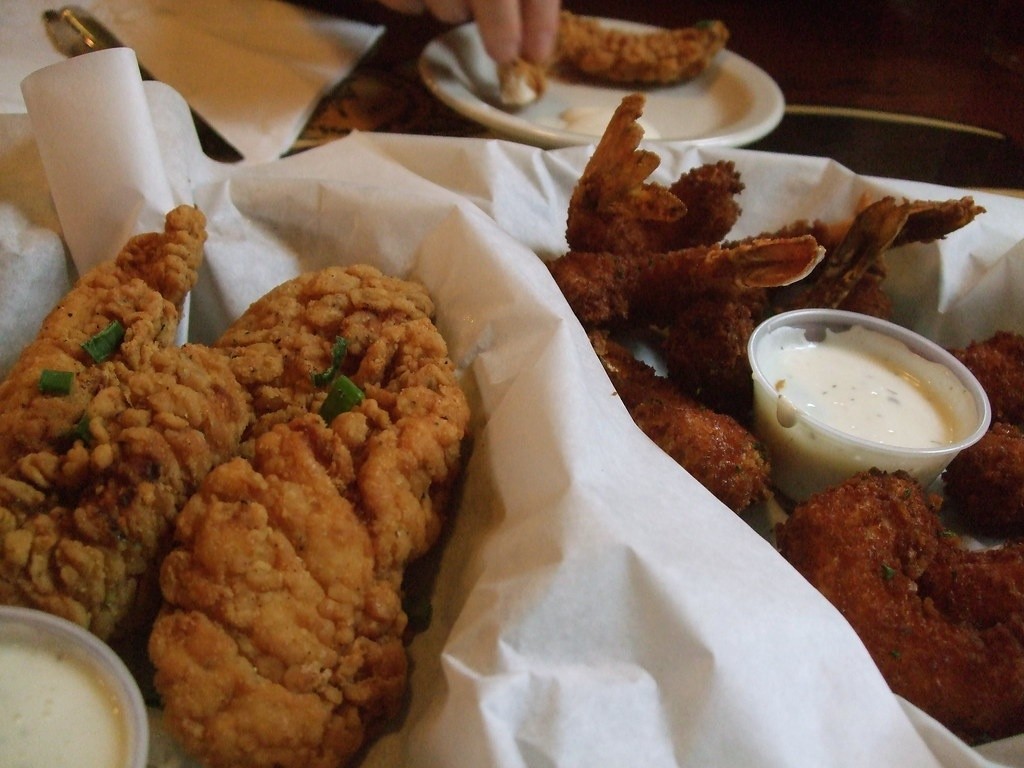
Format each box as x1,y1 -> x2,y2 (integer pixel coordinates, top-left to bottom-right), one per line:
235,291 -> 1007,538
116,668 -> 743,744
314,336 -> 362,422
36,319 -> 125,502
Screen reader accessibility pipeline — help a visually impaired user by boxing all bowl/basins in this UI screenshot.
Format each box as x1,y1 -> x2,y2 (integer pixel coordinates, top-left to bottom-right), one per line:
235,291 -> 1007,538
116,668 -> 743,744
747,307 -> 992,507
1,605 -> 149,768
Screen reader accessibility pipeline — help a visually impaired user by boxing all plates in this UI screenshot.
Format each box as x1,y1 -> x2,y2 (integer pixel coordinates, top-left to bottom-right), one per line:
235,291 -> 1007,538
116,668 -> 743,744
417,16 -> 784,149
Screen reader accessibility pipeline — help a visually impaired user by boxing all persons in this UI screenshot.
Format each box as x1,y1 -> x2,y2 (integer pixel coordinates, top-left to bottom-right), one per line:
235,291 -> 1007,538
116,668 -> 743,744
384,0 -> 560,65
